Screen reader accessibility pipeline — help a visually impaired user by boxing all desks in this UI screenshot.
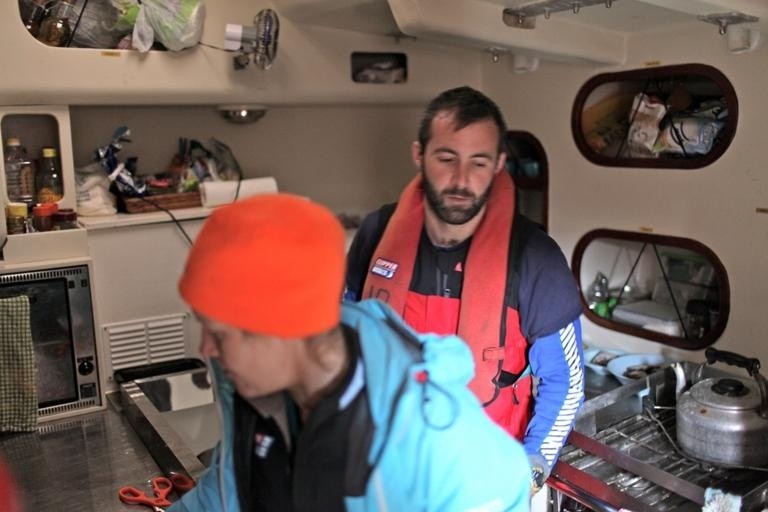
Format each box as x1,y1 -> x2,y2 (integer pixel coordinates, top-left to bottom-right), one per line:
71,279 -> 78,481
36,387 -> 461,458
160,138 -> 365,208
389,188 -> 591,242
610,287 -> 651,302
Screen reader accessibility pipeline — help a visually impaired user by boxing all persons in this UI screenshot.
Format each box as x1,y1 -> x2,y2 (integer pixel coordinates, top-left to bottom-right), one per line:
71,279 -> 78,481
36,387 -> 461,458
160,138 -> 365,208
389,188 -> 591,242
163,194 -> 532,512
346,85 -> 586,498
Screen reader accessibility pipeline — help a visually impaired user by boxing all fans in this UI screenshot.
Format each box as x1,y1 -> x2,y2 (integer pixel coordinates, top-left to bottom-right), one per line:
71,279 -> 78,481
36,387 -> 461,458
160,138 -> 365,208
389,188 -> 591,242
224,8 -> 280,70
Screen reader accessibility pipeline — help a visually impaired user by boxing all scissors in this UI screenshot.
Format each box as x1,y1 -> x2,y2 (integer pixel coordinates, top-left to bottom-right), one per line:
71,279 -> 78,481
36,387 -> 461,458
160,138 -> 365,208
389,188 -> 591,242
169,470 -> 194,490
118,477 -> 172,512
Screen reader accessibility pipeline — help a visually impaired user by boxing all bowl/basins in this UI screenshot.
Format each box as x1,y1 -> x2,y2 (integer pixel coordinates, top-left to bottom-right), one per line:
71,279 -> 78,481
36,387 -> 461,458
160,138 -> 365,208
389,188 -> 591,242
607,353 -> 666,396
583,346 -> 626,376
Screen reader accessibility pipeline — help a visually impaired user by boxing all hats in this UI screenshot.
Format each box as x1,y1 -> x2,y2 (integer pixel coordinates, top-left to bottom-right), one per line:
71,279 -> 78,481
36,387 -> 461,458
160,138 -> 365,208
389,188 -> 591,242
178,194 -> 345,338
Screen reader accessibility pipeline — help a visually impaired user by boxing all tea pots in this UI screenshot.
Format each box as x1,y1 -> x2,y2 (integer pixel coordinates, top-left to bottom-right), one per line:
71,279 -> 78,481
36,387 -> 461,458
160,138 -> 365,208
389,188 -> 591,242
669,346 -> 768,465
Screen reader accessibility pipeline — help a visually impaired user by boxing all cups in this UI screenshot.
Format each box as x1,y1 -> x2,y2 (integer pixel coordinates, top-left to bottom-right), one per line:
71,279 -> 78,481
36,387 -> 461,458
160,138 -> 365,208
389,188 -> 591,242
25,3 -> 44,38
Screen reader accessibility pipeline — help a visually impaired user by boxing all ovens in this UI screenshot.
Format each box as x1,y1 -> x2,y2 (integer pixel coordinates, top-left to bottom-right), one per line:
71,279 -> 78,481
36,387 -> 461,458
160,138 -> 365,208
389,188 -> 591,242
0,264 -> 102,426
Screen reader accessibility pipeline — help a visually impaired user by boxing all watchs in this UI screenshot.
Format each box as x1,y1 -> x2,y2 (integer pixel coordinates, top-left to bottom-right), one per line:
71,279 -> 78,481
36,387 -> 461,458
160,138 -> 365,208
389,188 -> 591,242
530,467 -> 544,491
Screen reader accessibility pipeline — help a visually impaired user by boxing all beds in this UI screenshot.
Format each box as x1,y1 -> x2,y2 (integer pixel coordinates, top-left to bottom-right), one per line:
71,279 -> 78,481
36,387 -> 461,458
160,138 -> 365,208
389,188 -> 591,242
613,276 -> 708,337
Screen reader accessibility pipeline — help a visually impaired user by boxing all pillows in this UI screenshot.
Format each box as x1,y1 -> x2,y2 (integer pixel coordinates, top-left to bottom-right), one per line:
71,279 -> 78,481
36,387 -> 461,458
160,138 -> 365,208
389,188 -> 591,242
627,93 -> 671,151
653,117 -> 723,155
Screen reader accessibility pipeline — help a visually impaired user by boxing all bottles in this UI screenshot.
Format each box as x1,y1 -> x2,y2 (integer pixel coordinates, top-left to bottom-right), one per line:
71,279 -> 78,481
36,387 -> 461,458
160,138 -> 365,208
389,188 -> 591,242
46,0 -> 76,49
36,148 -> 63,203
5,138 -> 34,206
5,202 -> 78,235
